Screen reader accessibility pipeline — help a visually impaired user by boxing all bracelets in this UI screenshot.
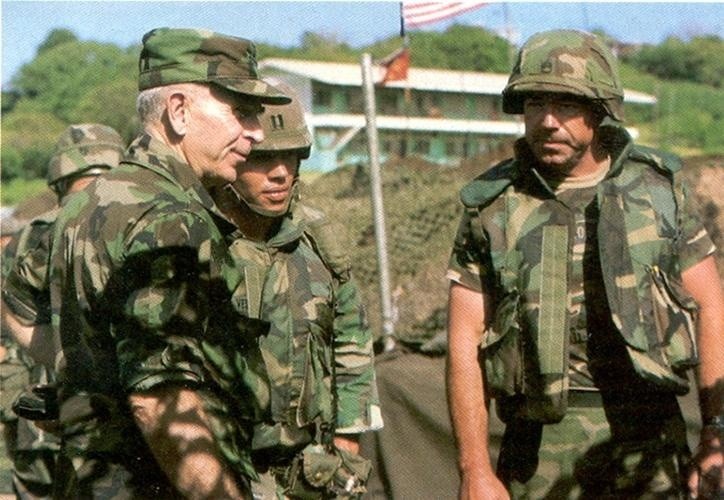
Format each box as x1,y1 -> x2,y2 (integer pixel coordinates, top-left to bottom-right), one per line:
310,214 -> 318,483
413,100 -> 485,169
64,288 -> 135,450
701,414 -> 724,427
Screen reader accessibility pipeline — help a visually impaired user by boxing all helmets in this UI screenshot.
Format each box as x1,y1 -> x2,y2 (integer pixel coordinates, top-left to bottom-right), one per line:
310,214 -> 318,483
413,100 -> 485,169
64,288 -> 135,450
500,28 -> 625,123
43,123 -> 126,190
251,73 -> 312,159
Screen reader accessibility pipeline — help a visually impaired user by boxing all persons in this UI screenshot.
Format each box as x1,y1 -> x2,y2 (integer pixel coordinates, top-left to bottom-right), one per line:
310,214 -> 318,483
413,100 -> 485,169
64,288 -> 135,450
204,81 -> 384,499
444,28 -> 724,500
45,27 -> 297,500
0,124 -> 127,500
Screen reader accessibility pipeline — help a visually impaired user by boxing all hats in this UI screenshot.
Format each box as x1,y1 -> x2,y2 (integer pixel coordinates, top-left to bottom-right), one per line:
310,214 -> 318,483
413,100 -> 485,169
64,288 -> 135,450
136,26 -> 292,106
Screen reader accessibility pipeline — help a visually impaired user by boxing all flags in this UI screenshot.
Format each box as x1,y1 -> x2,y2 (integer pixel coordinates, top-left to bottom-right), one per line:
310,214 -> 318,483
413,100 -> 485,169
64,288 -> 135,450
402,2 -> 488,30
374,45 -> 408,89
